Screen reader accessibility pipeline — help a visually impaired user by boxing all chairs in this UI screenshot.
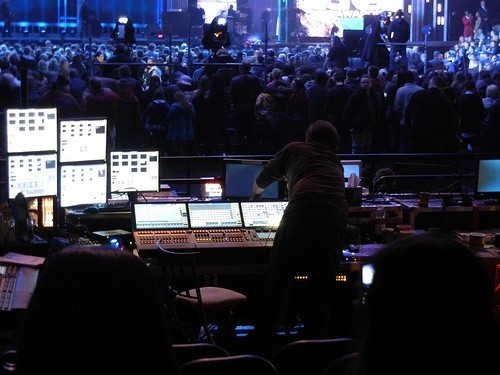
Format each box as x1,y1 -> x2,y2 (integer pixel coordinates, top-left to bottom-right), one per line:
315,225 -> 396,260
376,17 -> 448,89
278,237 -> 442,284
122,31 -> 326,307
157,246 -> 249,353
271,337 -> 361,375
13,246 -> 175,375
179,354 -> 277,375
358,230 -> 500,375
172,342 -> 231,366
322,351 -> 361,375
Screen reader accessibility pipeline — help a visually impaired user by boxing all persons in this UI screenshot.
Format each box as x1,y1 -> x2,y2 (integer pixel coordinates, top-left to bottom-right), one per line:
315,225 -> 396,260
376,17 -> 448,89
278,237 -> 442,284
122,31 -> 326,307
0,0 -> 500,375
230,61 -> 262,154
344,75 -> 378,154
387,10 -> 410,72
85,76 -> 118,151
248,120 -> 349,353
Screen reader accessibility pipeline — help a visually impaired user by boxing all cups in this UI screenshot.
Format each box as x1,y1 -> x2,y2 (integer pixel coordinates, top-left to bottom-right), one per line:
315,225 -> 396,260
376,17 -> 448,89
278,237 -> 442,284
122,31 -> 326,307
470,235 -> 485,250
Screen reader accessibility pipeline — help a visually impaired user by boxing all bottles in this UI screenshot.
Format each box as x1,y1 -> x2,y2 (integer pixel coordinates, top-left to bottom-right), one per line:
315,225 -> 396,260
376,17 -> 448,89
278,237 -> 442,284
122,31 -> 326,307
374,207 -> 386,236
418,192 -> 430,208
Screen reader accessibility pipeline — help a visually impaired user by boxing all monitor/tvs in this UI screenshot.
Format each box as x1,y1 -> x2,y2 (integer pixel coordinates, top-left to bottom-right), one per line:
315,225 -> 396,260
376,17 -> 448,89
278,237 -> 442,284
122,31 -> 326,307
4,106 -> 291,232
339,160 -> 362,188
474,157 -> 500,205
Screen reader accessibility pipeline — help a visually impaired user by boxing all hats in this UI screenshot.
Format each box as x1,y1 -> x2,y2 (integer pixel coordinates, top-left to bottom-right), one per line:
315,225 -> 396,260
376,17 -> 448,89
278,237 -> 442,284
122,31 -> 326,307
178,75 -> 192,85
254,93 -> 275,117
396,9 -> 403,15
